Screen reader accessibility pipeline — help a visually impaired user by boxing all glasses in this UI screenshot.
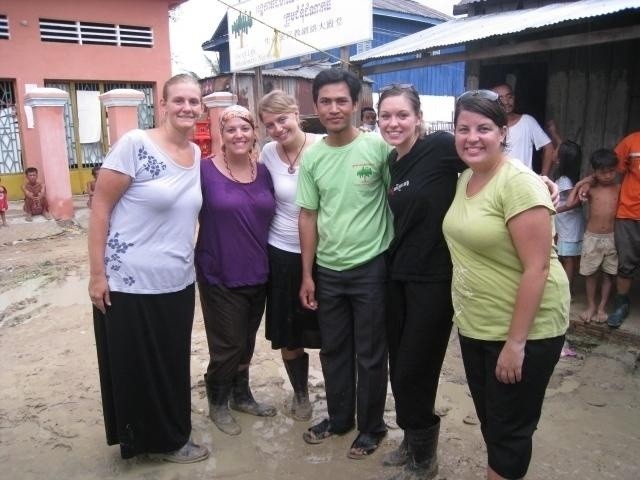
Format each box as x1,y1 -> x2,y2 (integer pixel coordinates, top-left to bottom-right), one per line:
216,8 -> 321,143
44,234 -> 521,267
377,82 -> 413,100
455,89 -> 500,103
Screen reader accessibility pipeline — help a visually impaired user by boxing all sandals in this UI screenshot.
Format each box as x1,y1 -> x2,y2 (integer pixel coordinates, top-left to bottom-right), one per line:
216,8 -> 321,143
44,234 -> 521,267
303,419 -> 355,443
347,430 -> 388,459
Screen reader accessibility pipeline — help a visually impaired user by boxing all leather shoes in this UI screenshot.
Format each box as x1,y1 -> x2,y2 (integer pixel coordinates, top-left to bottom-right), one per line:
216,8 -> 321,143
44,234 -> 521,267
149,443 -> 208,464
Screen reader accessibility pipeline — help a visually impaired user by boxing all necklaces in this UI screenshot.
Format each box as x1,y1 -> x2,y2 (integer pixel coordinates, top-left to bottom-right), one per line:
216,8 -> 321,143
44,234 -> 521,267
282,132 -> 308,173
221,150 -> 255,184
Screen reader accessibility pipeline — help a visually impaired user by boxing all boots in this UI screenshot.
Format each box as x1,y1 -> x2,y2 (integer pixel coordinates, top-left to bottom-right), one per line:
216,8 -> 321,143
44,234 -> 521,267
607,293 -> 630,327
230,367 -> 276,416
380,437 -> 408,467
387,416 -> 440,479
284,352 -> 312,420
204,373 -> 241,435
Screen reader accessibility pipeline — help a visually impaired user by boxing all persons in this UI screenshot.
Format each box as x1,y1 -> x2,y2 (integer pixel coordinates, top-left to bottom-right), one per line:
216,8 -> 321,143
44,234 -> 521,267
199,103 -> 279,437
85,71 -> 209,464
20,166 -> 52,222
440,88 -> 573,479
86,167 -> 102,208
359,105 -> 377,132
375,84 -> 563,478
292,67 -> 391,460
0,179 -> 9,229
255,90 -> 330,423
492,79 -> 640,330
